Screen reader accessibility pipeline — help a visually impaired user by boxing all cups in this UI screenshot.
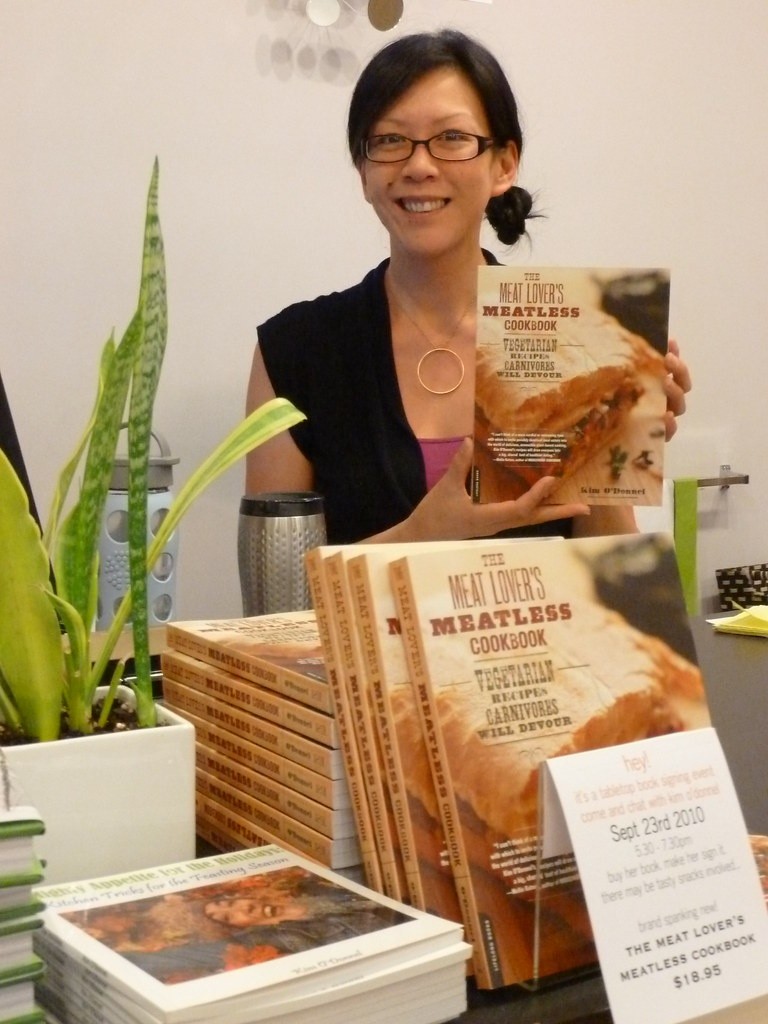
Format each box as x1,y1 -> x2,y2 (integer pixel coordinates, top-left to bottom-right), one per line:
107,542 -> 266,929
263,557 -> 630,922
238,490 -> 327,618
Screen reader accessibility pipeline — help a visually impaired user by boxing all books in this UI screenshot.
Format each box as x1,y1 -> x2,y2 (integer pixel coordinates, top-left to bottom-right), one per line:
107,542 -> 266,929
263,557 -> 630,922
0,533 -> 719,1024
468,264 -> 672,507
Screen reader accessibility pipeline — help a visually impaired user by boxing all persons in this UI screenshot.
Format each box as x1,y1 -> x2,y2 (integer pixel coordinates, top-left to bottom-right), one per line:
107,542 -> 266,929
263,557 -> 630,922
242,27 -> 692,544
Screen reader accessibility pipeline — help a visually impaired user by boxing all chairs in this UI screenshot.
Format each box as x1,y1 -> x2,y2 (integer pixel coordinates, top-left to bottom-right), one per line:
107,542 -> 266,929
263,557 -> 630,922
632,479 -> 705,619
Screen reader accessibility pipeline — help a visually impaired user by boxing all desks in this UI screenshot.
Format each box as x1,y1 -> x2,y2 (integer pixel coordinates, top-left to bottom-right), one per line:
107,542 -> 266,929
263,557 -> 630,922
458,605 -> 768,1024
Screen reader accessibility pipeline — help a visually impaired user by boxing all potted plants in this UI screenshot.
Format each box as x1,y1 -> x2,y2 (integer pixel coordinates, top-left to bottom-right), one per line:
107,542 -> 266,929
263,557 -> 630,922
0,156 -> 306,890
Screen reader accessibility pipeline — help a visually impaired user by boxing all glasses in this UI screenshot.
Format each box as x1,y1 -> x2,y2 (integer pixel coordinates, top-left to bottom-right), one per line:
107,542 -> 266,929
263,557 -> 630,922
362,128 -> 494,165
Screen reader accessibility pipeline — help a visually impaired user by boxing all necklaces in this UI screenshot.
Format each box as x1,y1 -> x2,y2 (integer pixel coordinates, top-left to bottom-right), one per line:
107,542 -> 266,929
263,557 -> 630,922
386,279 -> 479,393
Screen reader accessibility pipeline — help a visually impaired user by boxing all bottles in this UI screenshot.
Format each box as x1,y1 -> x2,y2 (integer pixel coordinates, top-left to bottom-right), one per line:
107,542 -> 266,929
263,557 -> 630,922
94,422 -> 180,629
